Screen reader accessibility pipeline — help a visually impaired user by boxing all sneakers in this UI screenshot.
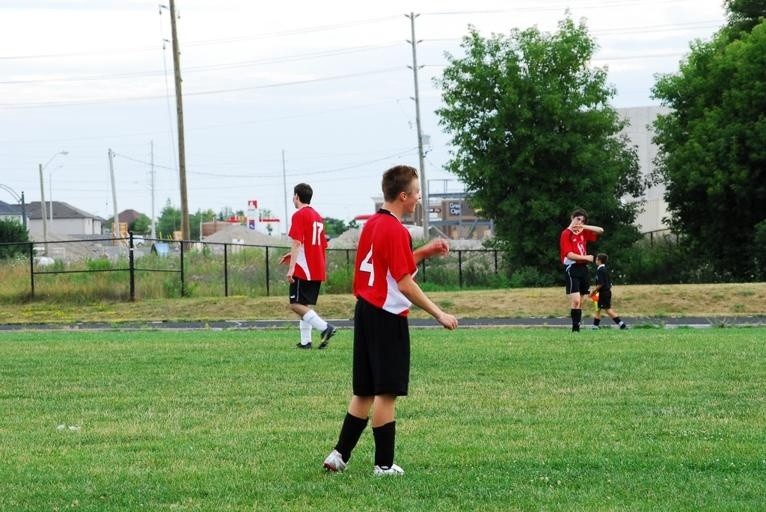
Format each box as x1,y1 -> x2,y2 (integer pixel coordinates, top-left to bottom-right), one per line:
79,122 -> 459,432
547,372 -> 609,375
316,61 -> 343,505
317,322 -> 337,349
292,342 -> 312,351
372,463 -> 405,478
323,449 -> 347,474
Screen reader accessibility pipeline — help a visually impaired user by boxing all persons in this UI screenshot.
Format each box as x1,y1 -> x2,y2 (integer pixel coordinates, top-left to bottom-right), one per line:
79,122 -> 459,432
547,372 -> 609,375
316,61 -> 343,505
587,253 -> 629,330
321,165 -> 461,476
277,182 -> 338,350
560,207 -> 605,333
